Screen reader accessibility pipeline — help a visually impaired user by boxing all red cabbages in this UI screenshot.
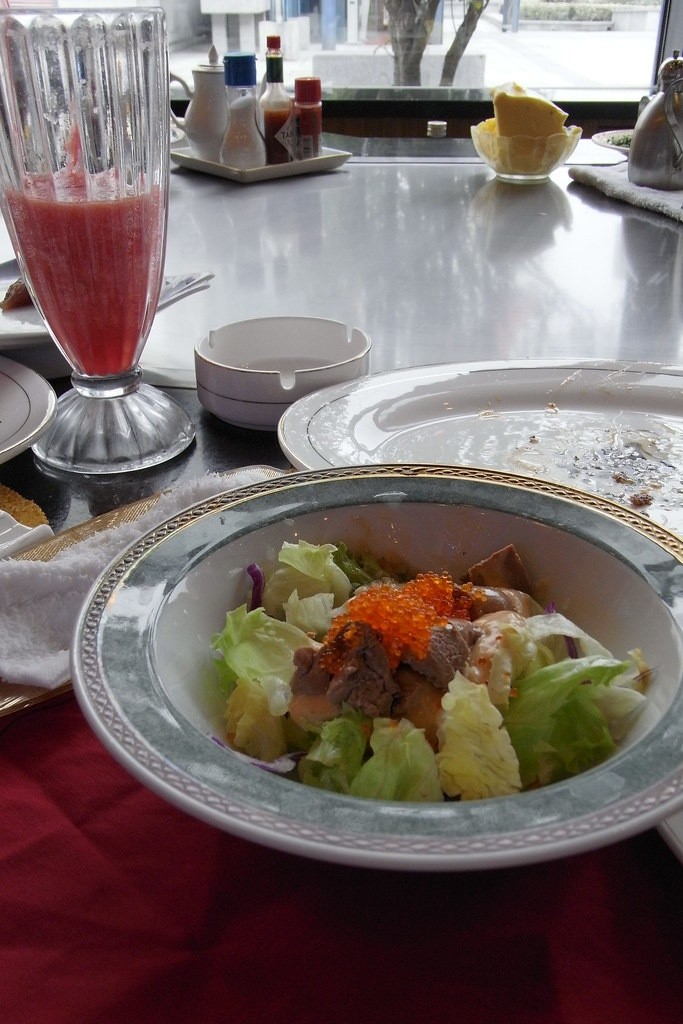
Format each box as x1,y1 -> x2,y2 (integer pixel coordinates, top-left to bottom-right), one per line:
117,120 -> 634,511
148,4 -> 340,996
246,563 -> 265,612
547,601 -> 578,659
207,734 -> 309,776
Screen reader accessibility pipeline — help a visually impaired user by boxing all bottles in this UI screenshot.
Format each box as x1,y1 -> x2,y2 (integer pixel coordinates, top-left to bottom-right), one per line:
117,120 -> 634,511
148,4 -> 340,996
219,53 -> 269,171
261,36 -> 296,165
292,76 -> 327,162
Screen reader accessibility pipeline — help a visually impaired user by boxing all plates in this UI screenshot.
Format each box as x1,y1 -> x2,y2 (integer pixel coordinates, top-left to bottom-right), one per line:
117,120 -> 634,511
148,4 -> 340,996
72,464 -> 683,869
0,251 -> 68,349
591,129 -> 633,157
166,148 -> 352,184
277,356 -> 683,548
0,354 -> 59,467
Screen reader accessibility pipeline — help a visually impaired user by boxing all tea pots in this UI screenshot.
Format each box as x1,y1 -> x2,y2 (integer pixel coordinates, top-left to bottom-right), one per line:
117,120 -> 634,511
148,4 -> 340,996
627,49 -> 683,191
166,43 -> 271,162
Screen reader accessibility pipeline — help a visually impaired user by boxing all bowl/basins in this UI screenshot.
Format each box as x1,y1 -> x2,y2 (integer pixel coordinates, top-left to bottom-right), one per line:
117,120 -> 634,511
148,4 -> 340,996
193,315 -> 373,434
470,125 -> 582,184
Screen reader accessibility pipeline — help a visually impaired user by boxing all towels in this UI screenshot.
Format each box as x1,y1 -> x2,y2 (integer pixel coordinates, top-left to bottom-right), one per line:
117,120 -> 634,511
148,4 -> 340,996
0,466 -> 275,691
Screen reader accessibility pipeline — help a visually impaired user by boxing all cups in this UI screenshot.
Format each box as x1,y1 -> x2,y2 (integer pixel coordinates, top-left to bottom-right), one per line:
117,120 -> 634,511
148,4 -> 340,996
0,1 -> 200,477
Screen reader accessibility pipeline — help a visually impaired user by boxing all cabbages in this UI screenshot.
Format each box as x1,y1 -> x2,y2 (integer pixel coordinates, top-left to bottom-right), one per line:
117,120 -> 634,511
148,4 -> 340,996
209,540 -> 647,804
